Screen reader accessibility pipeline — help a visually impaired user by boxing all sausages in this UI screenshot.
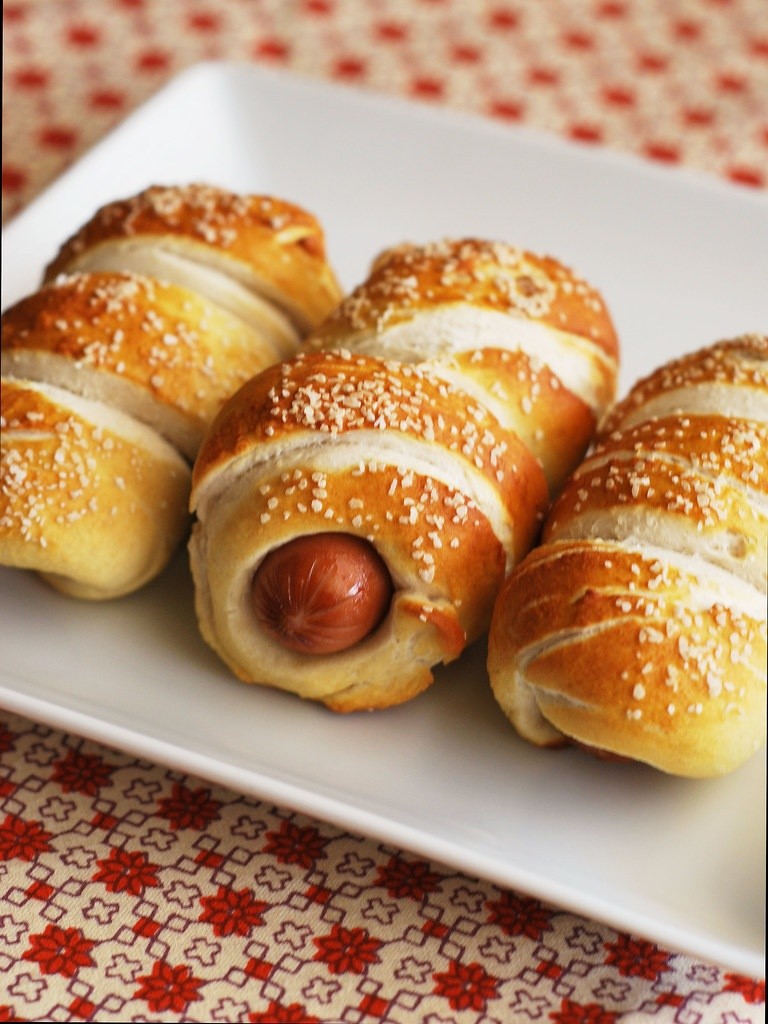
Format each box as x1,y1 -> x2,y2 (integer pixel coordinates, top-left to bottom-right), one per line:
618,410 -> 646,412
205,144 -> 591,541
248,531 -> 393,657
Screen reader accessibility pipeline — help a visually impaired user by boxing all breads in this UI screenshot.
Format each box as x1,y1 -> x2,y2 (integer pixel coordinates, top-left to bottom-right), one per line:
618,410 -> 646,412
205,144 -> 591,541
0,184 -> 768,779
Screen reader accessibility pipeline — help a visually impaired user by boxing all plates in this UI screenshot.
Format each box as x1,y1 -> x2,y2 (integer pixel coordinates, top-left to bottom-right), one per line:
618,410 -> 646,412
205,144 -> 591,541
0,58 -> 768,981
